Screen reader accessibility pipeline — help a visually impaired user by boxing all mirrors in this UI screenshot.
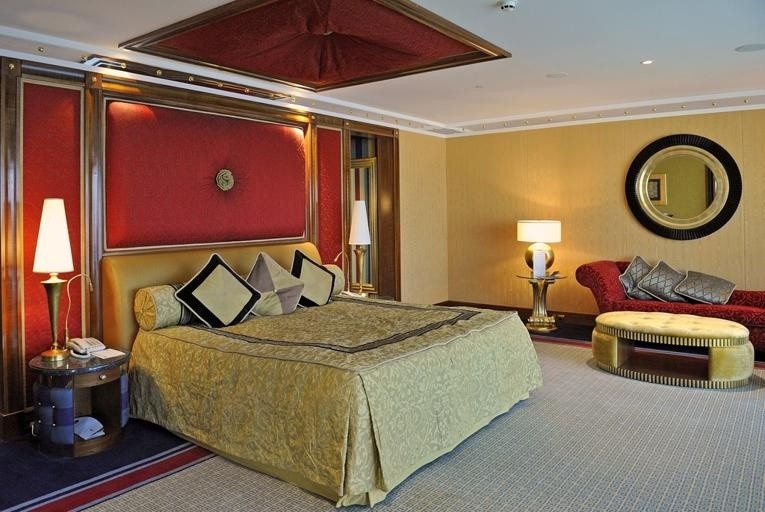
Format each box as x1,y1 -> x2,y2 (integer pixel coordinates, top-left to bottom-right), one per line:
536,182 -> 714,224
625,134 -> 744,242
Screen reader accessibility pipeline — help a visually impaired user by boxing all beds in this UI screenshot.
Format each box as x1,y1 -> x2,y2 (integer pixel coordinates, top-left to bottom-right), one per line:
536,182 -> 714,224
101,239 -> 545,511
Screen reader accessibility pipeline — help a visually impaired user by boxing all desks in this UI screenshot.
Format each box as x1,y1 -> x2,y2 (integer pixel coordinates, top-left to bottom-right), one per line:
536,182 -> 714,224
516,273 -> 569,336
356,292 -> 395,302
591,310 -> 756,389
27,346 -> 132,460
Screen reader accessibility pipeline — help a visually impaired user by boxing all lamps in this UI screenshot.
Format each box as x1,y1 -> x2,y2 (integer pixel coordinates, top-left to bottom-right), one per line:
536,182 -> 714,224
347,199 -> 373,296
516,218 -> 562,278
32,197 -> 76,365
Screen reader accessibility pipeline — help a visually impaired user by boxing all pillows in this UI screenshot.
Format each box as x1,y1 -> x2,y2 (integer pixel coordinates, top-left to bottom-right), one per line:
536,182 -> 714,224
173,252 -> 262,330
619,255 -> 655,301
637,260 -> 689,303
245,252 -> 305,318
134,263 -> 344,331
290,247 -> 337,310
673,269 -> 737,305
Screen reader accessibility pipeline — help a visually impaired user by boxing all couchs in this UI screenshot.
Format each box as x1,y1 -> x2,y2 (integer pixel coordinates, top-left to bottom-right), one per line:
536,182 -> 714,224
575,260 -> 764,360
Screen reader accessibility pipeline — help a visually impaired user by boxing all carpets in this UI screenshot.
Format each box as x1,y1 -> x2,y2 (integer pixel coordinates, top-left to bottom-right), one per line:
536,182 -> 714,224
2,331 -> 765,511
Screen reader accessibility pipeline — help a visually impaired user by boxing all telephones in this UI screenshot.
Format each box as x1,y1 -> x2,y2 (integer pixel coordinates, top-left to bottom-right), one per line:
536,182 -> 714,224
67,337 -> 106,355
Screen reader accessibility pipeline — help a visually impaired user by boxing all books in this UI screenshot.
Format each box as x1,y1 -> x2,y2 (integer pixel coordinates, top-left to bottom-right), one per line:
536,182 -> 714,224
74,416 -> 106,441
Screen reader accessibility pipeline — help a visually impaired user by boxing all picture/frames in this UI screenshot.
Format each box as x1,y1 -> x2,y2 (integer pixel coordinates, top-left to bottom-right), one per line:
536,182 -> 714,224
647,172 -> 668,207
705,164 -> 719,211
